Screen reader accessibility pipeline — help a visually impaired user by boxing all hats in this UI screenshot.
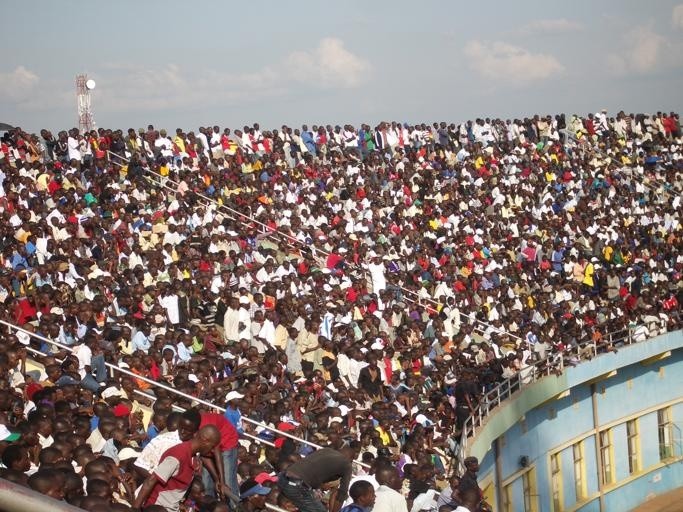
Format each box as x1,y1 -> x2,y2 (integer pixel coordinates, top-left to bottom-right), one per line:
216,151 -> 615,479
255,472 -> 279,485
223,390 -> 246,403
160,129 -> 167,135
372,343 -> 384,350
15,330 -> 31,346
58,376 -> 80,388
117,448 -> 143,461
220,352 -> 236,360
189,374 -> 201,383
309,266 -> 344,293
240,484 -> 272,499
325,295 -> 374,309
590,256 -> 674,274
0,423 -> 20,443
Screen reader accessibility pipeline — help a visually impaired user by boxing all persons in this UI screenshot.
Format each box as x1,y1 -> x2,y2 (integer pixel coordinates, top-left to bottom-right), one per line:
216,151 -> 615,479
0,107 -> 683,512
